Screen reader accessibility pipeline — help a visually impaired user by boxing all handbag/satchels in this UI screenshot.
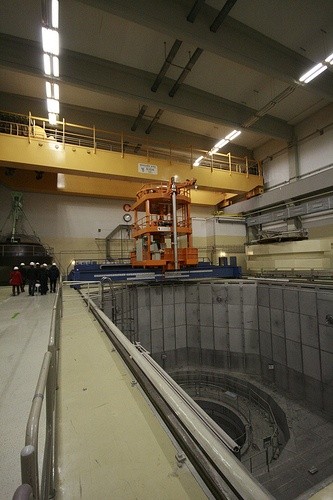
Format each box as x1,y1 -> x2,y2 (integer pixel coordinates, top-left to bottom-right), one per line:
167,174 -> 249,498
9,279 -> 13,285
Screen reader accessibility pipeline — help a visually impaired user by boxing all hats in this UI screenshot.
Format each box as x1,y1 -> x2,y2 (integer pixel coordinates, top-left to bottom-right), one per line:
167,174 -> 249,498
30,262 -> 35,265
43,264 -> 48,267
36,262 -> 39,264
14,267 -> 19,270
52,263 -> 56,266
20,263 -> 25,266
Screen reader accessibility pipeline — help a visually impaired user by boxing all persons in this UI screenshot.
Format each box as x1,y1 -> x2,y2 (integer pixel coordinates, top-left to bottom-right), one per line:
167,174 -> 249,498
10,261 -> 60,296
